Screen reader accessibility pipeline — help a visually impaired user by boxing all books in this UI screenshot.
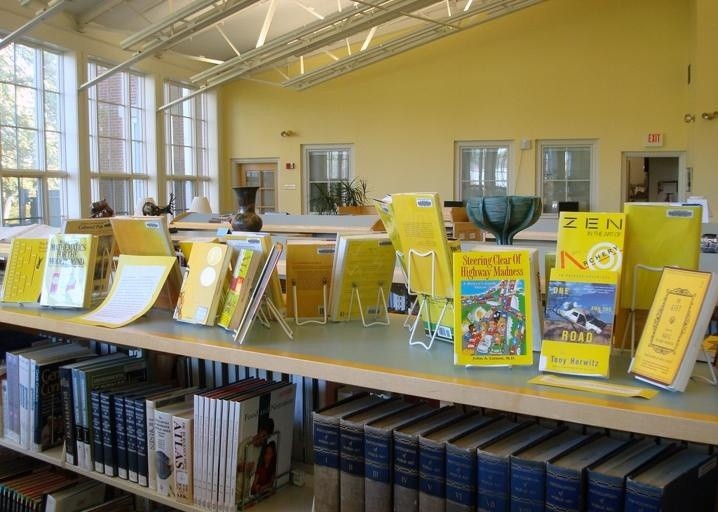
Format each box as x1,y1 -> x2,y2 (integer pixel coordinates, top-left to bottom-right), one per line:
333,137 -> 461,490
413,291 -> 454,343
330,232 -> 394,326
1,345 -> 149,486
466,241 -> 543,350
0,223 -> 63,258
175,233 -> 218,263
62,216 -> 117,295
445,247 -> 534,369
555,208 -> 631,276
616,197 -> 706,309
222,230 -> 284,321
283,237 -> 335,320
537,265 -> 620,378
0,470 -> 125,512
213,248 -> 259,333
390,193 -> 458,298
174,240 -> 233,327
310,399 -> 718,512
231,237 -> 285,348
108,214 -> 186,312
40,232 -> 96,309
624,265 -> 712,391
2,236 -> 47,305
150,376 -> 295,505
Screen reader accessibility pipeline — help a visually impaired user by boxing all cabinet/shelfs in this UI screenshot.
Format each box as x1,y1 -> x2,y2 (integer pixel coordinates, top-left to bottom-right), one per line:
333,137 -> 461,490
0,295 -> 718,512
169,212 -> 423,316
338,204 -> 484,243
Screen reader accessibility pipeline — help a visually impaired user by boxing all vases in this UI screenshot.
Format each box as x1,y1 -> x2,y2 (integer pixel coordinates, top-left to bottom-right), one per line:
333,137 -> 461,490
228,184 -> 267,234
